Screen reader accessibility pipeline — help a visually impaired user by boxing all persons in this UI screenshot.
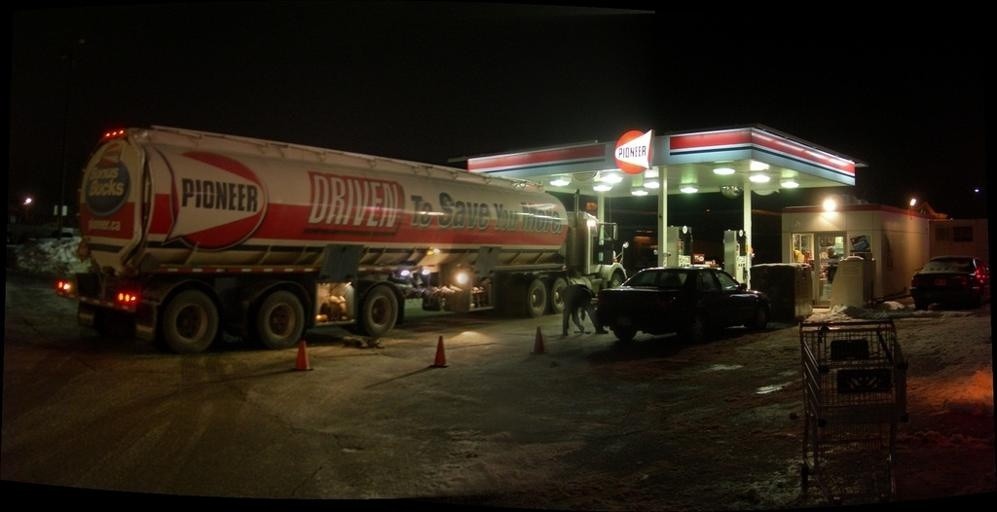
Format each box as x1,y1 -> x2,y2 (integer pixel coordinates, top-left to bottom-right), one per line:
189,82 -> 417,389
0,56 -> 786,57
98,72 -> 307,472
559,283 -> 608,338
823,246 -> 839,283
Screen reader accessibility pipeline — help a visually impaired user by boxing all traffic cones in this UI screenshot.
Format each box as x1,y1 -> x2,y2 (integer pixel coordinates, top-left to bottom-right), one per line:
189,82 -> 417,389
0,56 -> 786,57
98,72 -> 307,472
288,336 -> 316,373
427,335 -> 450,368
529,324 -> 547,356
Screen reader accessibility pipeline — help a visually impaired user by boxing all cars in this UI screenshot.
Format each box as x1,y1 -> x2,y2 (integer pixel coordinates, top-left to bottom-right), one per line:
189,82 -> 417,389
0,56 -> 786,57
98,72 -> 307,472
909,253 -> 990,312
592,265 -> 774,346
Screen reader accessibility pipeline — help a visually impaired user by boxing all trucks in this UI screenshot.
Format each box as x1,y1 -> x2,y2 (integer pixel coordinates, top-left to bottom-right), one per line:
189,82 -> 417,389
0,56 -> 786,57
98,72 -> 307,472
48,117 -> 631,359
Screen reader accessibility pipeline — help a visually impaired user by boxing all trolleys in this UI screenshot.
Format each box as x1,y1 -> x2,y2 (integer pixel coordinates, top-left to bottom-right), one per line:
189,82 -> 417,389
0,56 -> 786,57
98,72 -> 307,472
794,316 -> 909,512
